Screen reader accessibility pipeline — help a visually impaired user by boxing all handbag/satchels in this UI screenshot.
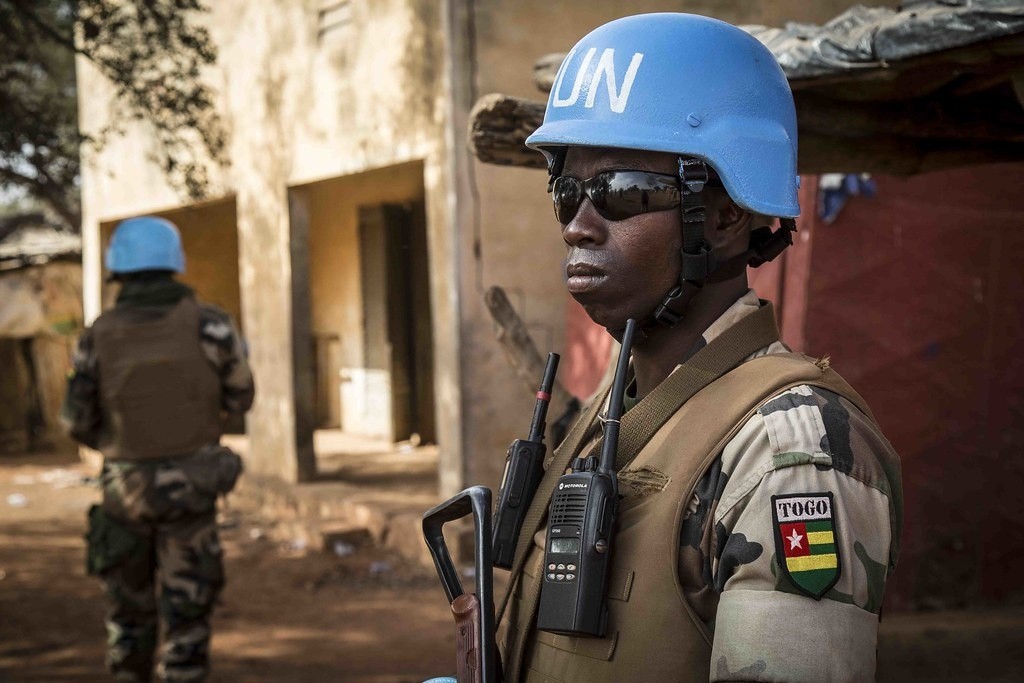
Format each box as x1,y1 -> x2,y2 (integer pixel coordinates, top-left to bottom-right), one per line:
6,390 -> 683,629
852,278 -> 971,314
181,444 -> 243,494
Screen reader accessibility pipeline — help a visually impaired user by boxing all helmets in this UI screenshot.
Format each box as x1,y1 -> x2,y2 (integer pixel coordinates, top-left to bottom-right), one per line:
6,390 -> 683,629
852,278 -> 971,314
106,216 -> 183,273
524,12 -> 802,219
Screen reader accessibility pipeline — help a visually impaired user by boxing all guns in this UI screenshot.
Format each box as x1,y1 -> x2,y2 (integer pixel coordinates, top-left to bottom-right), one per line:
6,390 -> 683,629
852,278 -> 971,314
418,483 -> 504,683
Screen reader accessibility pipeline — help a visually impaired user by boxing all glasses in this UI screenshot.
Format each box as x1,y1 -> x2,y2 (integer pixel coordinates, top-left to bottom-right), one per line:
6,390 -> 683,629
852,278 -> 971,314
552,168 -> 681,223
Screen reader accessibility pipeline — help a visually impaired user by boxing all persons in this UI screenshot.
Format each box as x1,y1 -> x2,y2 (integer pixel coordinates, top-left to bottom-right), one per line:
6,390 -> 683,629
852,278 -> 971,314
65,218 -> 255,683
502,12 -> 905,683
